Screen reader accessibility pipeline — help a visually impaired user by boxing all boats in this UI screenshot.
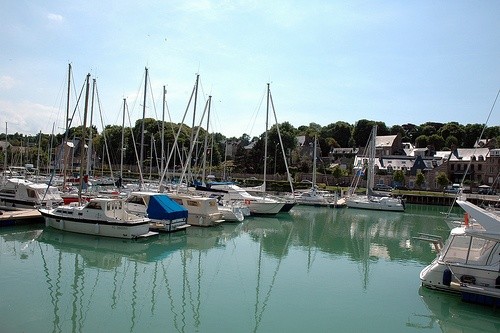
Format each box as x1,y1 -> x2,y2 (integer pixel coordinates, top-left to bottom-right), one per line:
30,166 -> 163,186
411,81 -> 500,299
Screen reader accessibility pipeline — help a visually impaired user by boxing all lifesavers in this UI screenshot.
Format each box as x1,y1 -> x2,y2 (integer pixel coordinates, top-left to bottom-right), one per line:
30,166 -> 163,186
460,275 -> 476,283
245,200 -> 250,204
465,211 -> 468,225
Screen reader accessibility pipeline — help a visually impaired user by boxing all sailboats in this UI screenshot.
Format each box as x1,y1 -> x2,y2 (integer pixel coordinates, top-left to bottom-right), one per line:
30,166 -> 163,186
37,73 -> 152,241
0,61 -> 251,232
284,131 -> 341,207
248,82 -> 299,212
347,125 -> 407,213
